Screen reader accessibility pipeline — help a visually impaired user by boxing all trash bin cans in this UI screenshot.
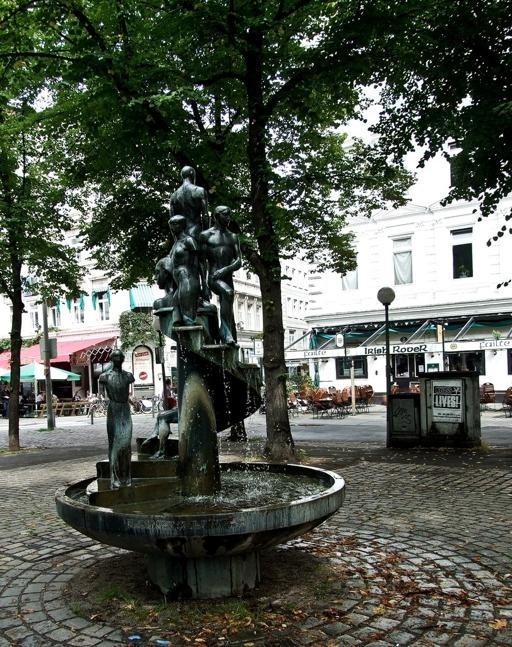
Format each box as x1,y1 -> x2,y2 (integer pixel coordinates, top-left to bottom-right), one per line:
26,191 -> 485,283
389,393 -> 421,449
417,370 -> 481,448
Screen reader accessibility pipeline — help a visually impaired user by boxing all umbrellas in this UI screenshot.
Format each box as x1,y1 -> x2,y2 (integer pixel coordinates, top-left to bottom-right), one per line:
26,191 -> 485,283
1,358 -> 82,407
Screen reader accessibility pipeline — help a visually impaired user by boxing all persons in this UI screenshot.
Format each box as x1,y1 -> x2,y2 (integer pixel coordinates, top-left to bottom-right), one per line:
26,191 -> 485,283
98,348 -> 135,489
161,378 -> 178,410
198,204 -> 241,346
168,213 -> 220,327
37,391 -> 45,410
152,257 -> 177,311
0,384 -> 10,418
170,165 -> 213,305
71,390 -> 82,401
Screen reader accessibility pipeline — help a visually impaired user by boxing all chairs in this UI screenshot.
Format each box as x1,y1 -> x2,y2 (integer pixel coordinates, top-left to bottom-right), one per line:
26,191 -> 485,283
288,384 -> 374,417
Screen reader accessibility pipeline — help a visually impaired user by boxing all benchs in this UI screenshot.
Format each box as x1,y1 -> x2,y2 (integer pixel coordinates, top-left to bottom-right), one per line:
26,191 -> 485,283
37,399 -> 110,417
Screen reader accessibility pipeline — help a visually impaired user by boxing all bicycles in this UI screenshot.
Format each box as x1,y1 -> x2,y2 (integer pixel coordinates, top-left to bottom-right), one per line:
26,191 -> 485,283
88,394 -> 167,418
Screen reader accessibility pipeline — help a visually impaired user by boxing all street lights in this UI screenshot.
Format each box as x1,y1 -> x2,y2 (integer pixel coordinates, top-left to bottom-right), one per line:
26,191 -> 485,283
151,316 -> 173,437
377,285 -> 397,448
